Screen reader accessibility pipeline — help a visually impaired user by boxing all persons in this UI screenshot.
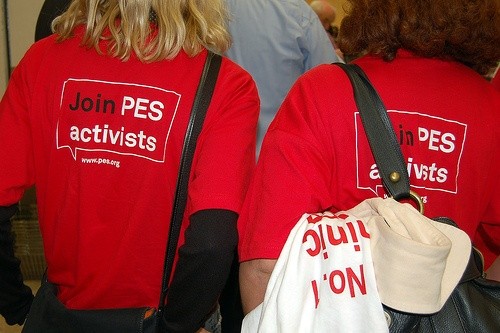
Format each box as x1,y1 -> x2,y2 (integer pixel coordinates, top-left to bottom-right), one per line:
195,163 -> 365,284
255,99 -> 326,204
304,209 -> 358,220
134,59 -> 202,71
0,0 -> 260,333
236,0 -> 500,333
218,0 -> 345,163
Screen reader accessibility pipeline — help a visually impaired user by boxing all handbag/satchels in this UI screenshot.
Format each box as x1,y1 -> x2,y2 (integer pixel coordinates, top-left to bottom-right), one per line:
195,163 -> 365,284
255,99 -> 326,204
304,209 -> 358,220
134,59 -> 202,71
332,62 -> 500,333
21,281 -> 157,333
